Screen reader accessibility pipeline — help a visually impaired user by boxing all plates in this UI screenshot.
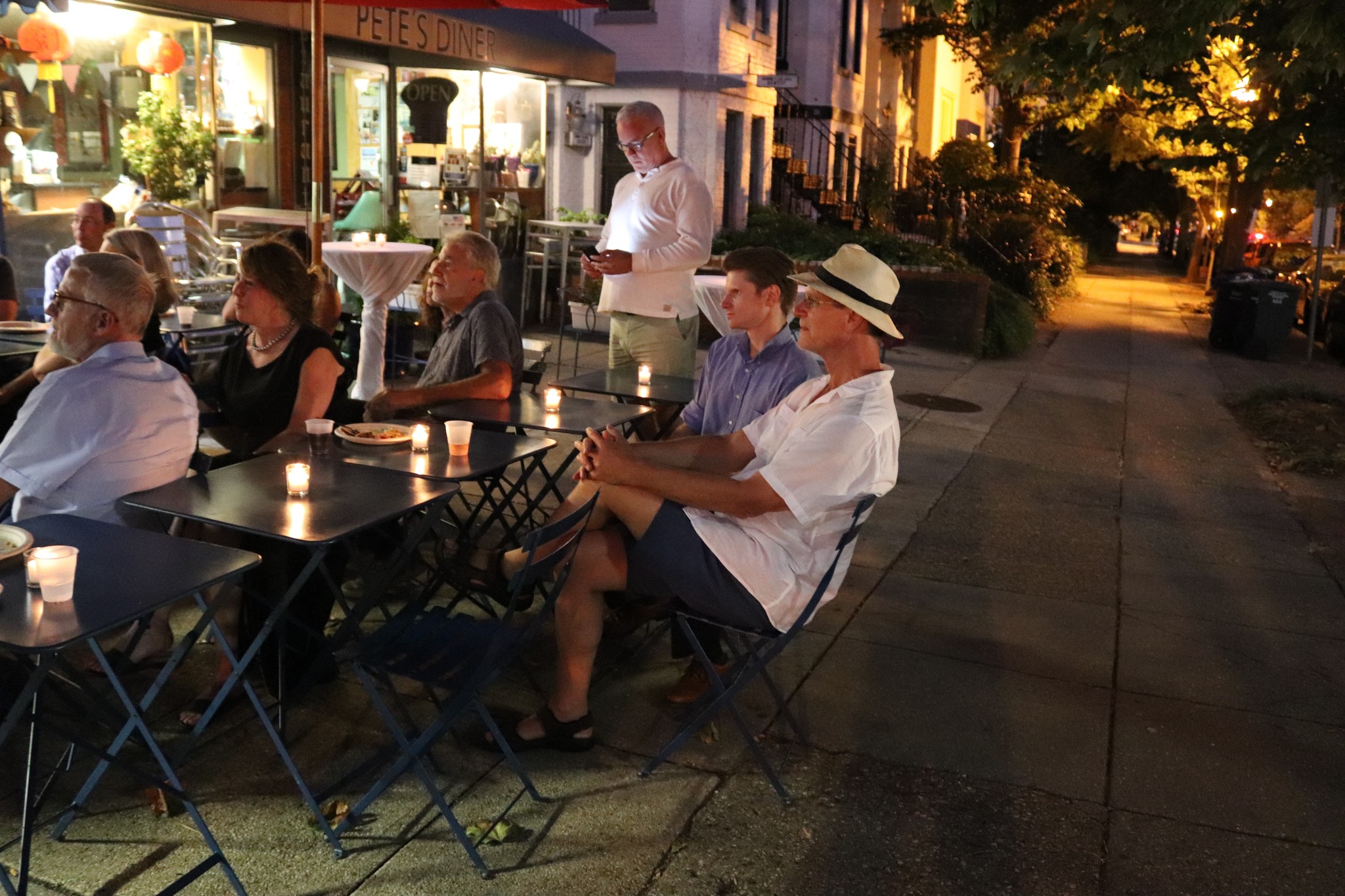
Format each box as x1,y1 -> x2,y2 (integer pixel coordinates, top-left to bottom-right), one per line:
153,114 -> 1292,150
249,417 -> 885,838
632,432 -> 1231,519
0,523 -> 34,560
334,423 -> 413,445
0,320 -> 49,331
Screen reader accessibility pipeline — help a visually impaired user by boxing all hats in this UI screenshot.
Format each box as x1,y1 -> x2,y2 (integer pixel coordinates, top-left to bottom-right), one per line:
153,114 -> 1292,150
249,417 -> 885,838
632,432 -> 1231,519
785,243 -> 904,340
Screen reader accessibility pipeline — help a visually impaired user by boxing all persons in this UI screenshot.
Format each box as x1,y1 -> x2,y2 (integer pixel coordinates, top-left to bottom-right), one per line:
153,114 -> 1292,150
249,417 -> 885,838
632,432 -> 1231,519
221,228 -> 341,336
342,230 -> 523,597
90,244 -> 355,729
580,101 -> 714,443
666,247 -> 823,712
0,197 -> 205,536
435,244 -> 903,753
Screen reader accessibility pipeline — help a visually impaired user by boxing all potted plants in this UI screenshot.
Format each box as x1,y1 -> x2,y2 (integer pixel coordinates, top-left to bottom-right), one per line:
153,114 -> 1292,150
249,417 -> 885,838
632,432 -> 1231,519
569,274 -> 610,333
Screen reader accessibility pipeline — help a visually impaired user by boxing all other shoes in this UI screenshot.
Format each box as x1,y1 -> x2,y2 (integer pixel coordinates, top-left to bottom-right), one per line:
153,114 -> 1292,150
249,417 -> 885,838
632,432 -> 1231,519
341,557 -> 413,599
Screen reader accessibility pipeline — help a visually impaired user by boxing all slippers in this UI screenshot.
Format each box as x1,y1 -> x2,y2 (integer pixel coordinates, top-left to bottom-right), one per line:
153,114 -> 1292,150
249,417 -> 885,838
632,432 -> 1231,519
83,644 -> 165,677
183,680 -> 247,736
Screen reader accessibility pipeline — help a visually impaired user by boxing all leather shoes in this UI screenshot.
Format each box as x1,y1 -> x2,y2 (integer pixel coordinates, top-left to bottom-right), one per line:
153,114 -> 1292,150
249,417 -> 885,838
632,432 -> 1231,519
664,650 -> 737,706
602,597 -> 671,638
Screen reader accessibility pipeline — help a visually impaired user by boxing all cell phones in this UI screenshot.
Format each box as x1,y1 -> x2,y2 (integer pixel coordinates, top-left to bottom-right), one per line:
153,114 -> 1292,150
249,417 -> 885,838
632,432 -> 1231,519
580,245 -> 601,262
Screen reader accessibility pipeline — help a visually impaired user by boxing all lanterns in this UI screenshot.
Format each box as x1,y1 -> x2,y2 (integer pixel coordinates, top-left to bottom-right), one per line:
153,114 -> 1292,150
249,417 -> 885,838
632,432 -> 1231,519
18,13 -> 72,113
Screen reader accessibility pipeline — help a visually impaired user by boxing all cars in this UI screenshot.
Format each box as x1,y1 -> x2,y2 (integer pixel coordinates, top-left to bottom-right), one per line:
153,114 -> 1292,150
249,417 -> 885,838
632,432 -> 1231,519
1242,237 -> 1345,359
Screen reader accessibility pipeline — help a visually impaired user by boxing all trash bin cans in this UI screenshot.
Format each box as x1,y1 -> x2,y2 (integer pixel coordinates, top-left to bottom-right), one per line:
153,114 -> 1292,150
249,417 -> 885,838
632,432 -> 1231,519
1208,278 -> 1303,360
1226,263 -> 1276,281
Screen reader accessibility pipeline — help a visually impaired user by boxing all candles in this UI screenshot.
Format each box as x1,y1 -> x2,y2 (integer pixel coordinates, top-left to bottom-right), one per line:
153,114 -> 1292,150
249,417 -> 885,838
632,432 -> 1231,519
288,463 -> 308,491
638,366 -> 650,384
412,424 -> 428,448
27,560 -> 39,584
546,389 -> 561,409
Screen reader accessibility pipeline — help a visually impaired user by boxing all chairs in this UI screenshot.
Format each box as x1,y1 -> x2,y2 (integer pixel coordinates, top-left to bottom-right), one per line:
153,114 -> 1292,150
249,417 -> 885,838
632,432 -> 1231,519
130,202 -> 243,290
522,337 -> 551,394
323,486 -> 602,880
165,255 -> 232,382
637,489 -> 877,808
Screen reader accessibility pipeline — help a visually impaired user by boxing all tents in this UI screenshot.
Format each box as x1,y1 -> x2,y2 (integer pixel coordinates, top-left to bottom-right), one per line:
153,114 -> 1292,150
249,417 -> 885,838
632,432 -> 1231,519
136,31 -> 185,78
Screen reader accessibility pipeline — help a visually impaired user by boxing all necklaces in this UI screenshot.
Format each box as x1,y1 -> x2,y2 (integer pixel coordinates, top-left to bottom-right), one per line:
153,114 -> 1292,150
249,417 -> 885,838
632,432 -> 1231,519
251,318 -> 298,350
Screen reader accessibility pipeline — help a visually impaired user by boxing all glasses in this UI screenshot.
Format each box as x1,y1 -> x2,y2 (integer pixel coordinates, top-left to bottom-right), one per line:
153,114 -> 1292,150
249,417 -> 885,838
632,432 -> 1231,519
615,126 -> 660,151
52,289 -> 119,323
68,216 -> 101,229
802,294 -> 839,309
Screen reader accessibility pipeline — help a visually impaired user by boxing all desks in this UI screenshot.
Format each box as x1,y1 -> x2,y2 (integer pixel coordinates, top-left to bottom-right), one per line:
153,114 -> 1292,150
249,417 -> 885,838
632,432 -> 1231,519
0,138 -> 804,896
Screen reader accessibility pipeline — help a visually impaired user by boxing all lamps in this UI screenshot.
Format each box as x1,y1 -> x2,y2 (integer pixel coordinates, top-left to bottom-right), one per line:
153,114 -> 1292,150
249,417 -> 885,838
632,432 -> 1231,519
566,92 -> 586,134
333,191 -> 385,241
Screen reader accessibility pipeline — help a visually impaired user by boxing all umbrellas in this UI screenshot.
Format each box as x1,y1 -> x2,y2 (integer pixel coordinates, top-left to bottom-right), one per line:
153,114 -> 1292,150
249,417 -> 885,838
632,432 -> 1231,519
234,0 -> 609,285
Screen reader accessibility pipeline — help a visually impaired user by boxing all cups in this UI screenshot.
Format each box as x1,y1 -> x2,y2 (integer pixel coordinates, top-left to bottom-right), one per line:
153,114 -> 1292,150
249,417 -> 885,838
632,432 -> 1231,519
23,547 -> 43,589
444,421 -> 474,456
176,306 -> 194,328
304,419 -> 334,456
285,463 -> 310,497
350,232 -> 387,247
638,365 -> 652,385
31,545 -> 80,601
543,388 -> 562,412
410,425 -> 431,452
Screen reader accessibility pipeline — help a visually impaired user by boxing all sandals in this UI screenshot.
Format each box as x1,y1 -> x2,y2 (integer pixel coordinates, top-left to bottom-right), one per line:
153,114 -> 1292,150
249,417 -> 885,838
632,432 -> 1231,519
433,537 -> 537,612
475,700 -> 597,754
